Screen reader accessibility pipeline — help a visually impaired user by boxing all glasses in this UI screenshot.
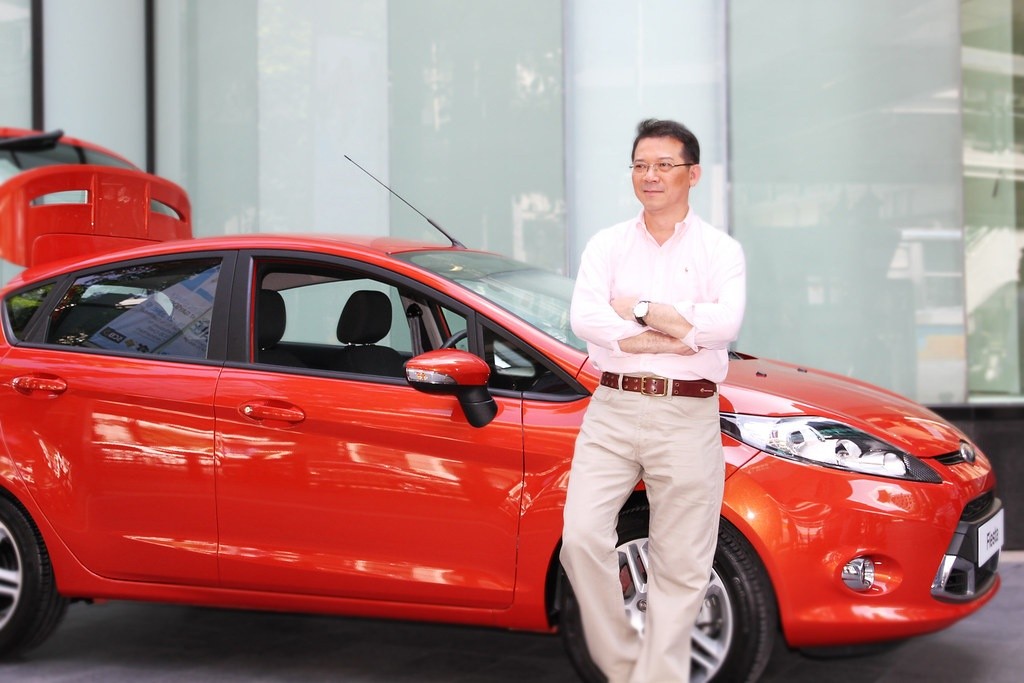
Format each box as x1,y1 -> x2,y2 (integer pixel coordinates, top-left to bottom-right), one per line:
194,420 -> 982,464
629,158 -> 694,177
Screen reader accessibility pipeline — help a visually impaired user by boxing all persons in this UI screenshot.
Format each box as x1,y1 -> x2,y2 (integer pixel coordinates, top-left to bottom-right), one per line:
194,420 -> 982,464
558,121 -> 746,683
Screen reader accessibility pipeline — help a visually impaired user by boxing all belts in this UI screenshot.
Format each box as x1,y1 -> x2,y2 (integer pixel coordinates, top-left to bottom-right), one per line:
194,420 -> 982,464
599,373 -> 717,398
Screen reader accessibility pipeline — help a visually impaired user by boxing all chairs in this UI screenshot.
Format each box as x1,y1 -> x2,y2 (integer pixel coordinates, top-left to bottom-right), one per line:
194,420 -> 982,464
335,290 -> 406,378
255,287 -> 302,370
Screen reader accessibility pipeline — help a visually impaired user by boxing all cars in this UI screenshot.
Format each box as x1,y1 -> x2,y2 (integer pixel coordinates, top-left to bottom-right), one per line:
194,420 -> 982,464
0,126 -> 1004,683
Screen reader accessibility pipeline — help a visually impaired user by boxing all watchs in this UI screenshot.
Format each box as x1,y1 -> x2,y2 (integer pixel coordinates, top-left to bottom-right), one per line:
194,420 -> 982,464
634,300 -> 652,326
